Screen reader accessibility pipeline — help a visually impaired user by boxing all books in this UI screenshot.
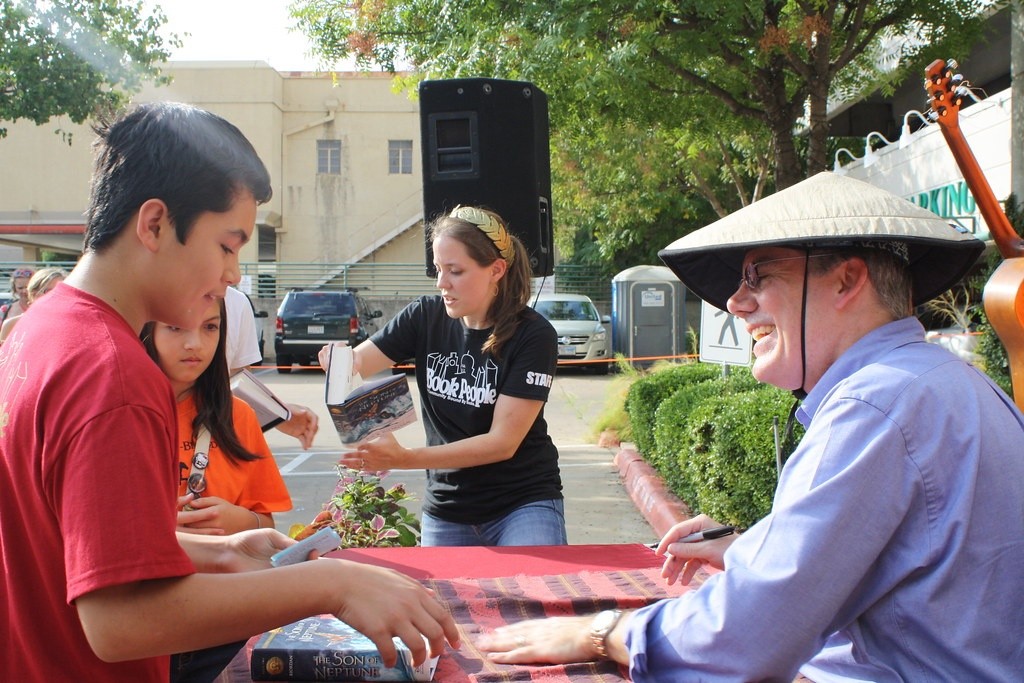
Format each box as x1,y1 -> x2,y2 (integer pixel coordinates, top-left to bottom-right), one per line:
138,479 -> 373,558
250,622 -> 440,683
230,368 -> 291,434
324,343 -> 418,450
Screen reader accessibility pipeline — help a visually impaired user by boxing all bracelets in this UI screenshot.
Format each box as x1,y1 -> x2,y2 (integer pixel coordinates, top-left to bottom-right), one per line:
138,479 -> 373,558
251,511 -> 260,529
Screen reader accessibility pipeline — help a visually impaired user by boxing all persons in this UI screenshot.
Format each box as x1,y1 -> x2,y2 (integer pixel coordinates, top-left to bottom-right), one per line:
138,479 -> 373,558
221,286 -> 319,450
139,297 -> 292,683
0,267 -> 65,347
320,206 -> 568,546
475,172 -> 1024,683
0,100 -> 461,683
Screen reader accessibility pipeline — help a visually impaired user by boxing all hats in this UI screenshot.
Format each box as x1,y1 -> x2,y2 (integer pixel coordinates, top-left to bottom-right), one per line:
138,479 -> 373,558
656,168 -> 988,315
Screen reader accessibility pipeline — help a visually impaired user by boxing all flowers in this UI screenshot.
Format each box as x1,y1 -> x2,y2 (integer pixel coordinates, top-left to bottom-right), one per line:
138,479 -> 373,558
286,456 -> 422,550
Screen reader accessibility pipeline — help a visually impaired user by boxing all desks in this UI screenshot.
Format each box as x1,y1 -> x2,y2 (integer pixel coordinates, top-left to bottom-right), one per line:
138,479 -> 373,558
212,544 -> 724,683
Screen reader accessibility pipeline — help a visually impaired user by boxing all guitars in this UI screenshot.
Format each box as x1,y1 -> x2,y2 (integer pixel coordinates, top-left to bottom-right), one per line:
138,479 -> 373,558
915,57 -> 1023,414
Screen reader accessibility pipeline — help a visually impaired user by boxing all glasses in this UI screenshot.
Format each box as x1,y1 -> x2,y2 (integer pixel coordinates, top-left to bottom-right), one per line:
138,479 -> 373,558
13,270 -> 34,278
737,252 -> 839,289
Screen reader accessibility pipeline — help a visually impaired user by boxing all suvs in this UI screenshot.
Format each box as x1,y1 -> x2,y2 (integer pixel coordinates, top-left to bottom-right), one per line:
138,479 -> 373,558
273,286 -> 385,375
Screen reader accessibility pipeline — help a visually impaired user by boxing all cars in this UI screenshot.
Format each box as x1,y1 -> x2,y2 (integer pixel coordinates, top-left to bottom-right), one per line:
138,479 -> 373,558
527,294 -> 612,374
243,293 -> 269,365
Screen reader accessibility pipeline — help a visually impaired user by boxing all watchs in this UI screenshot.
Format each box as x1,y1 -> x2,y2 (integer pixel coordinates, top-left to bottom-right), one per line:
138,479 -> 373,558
589,607 -> 623,657
